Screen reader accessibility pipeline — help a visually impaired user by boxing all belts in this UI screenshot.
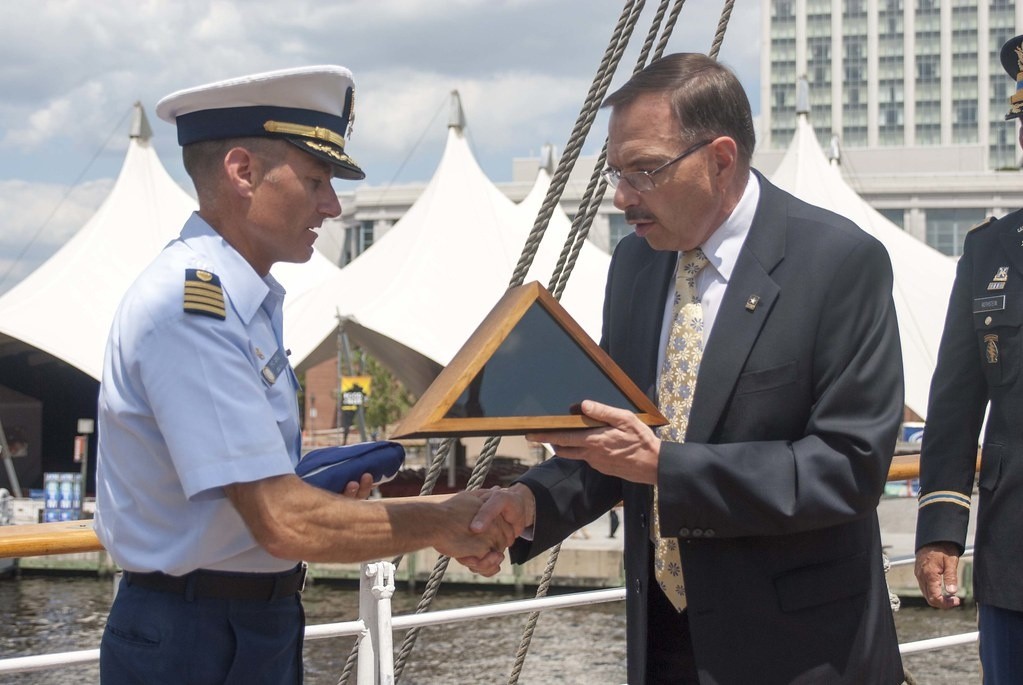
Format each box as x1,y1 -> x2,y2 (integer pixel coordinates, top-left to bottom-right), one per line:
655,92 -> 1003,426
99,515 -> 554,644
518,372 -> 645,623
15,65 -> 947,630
122,563 -> 308,597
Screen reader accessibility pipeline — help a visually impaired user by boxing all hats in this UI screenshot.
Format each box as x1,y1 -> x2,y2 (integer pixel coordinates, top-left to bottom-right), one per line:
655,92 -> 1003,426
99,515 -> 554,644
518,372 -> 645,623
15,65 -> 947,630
155,65 -> 367,181
999,35 -> 1023,119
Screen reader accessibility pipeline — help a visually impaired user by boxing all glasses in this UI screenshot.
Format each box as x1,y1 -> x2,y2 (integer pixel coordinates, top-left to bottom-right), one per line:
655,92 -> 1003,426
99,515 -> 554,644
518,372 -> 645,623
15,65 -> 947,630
600,140 -> 713,191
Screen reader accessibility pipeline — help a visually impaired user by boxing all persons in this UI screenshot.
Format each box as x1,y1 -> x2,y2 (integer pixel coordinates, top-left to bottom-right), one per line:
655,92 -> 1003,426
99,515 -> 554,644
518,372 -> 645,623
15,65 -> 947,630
94,65 -> 515,685
914,34 -> 1023,685
455,53 -> 906,685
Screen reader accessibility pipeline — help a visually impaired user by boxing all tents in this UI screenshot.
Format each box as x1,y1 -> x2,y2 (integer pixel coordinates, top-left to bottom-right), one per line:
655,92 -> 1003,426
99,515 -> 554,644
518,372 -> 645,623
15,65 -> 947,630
0,79 -> 957,423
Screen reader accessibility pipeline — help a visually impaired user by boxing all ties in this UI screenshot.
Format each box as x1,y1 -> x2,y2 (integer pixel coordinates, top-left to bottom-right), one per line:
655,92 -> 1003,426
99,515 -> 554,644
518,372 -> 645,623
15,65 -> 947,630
653,248 -> 710,613
